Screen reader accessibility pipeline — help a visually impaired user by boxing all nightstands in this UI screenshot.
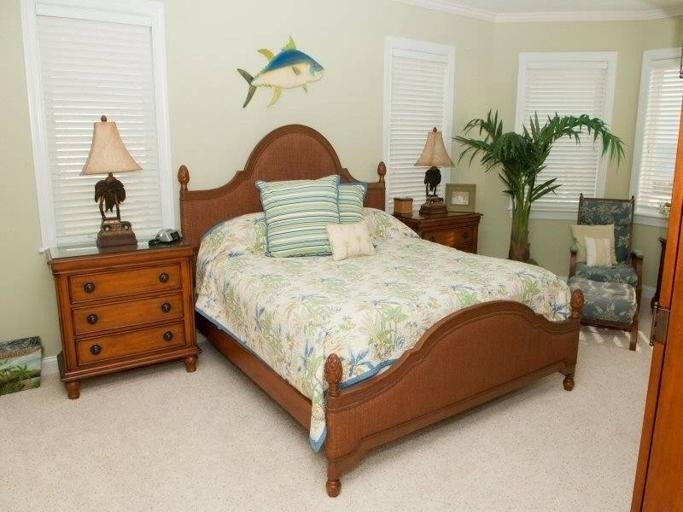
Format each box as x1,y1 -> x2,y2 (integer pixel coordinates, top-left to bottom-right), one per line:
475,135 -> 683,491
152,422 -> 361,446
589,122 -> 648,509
391,208 -> 484,255
44,238 -> 202,401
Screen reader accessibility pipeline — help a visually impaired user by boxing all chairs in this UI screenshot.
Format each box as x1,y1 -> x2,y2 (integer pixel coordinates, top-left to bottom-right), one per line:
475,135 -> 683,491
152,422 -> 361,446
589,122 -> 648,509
568,192 -> 646,353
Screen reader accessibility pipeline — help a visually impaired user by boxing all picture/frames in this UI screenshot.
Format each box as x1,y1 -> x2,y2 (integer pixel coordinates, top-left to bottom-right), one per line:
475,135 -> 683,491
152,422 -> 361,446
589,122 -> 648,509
444,182 -> 477,212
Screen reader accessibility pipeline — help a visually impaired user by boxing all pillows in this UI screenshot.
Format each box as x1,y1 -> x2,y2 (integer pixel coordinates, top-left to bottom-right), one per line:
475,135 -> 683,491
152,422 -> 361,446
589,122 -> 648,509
337,180 -> 367,225
326,218 -> 377,263
570,223 -> 619,269
250,173 -> 343,258
582,235 -> 613,268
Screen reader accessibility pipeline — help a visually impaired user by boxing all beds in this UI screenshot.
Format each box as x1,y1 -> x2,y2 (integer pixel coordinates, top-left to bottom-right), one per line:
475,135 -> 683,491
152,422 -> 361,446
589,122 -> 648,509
175,121 -> 588,499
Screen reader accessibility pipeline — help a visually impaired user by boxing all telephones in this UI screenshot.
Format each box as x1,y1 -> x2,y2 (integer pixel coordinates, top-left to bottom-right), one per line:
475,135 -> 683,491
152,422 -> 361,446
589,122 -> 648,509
155,227 -> 181,243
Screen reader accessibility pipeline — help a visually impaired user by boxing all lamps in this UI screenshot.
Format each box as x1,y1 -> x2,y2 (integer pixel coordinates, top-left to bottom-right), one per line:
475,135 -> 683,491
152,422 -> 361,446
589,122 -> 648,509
78,114 -> 143,245
413,126 -> 456,219
